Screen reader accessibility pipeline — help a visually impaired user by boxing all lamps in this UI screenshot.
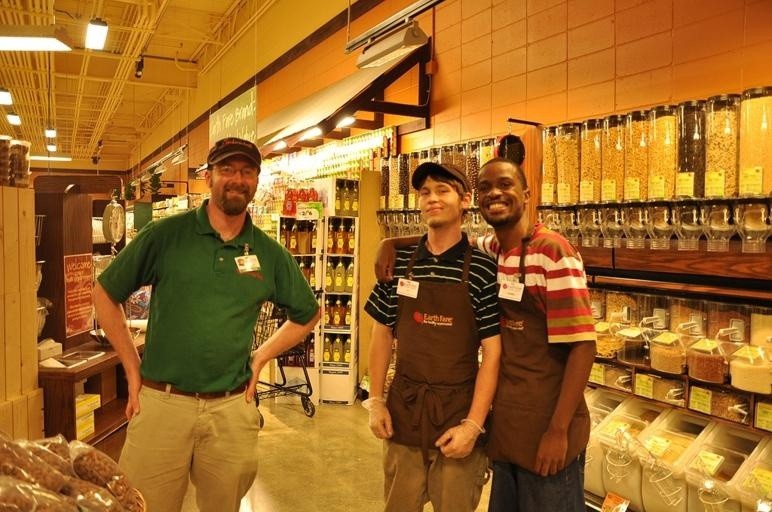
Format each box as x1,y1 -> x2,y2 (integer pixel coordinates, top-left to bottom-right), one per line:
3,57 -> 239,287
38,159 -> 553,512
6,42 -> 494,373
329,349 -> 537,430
140,173 -> 152,182
130,179 -> 140,186
43,121 -> 57,140
0,87 -> 13,108
44,143 -> 58,155
85,16 -> 109,52
4,112 -> 21,128
153,164 -> 167,175
170,153 -> 189,166
0,22 -> 74,54
356,19 -> 429,69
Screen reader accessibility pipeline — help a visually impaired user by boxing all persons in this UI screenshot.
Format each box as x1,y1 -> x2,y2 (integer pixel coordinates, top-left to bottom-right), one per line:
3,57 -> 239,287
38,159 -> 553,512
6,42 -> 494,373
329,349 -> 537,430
360,161 -> 504,512
374,156 -> 598,512
92,136 -> 321,512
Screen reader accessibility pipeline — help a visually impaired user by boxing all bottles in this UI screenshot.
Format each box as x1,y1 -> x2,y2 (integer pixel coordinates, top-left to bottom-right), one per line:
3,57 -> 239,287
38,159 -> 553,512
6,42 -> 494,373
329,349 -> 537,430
280,217 -> 314,255
324,256 -> 354,291
323,294 -> 352,332
322,333 -> 351,368
335,180 -> 358,217
326,217 -> 356,254
541,85 -> 768,252
378,134 -> 506,235
290,256 -> 314,289
278,334 -> 314,368
282,185 -> 317,216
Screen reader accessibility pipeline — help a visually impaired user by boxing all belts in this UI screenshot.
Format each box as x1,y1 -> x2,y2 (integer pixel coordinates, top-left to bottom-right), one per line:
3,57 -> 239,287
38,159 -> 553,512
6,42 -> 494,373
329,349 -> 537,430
139,378 -> 251,400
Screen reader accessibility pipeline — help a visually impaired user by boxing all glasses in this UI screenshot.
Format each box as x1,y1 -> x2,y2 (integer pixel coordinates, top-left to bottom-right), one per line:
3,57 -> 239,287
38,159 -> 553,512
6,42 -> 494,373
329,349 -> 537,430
210,165 -> 258,177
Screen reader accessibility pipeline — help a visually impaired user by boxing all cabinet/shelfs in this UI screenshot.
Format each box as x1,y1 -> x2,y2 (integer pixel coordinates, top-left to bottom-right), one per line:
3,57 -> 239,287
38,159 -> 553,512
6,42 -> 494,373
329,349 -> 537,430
38,328 -> 145,447
321,216 -> 362,407
275,216 -> 322,408
535,196 -> 772,512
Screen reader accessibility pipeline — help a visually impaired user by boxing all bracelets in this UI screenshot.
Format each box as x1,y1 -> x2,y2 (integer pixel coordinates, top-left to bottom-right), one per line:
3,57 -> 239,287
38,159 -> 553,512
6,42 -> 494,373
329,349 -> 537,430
460,417 -> 486,436
363,396 -> 388,409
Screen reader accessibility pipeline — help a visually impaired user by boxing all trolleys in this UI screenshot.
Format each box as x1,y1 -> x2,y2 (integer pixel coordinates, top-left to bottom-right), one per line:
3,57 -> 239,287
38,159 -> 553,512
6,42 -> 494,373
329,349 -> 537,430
246,289 -> 323,427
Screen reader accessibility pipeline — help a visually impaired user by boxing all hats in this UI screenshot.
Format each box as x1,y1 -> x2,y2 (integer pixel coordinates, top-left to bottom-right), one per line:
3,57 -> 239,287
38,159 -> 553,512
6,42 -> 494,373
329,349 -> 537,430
411,160 -> 472,192
206,136 -> 262,173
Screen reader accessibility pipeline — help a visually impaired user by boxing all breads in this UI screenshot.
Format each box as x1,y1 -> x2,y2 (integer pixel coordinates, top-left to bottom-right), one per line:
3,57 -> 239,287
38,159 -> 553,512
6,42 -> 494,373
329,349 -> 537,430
0,432 -> 141,512
